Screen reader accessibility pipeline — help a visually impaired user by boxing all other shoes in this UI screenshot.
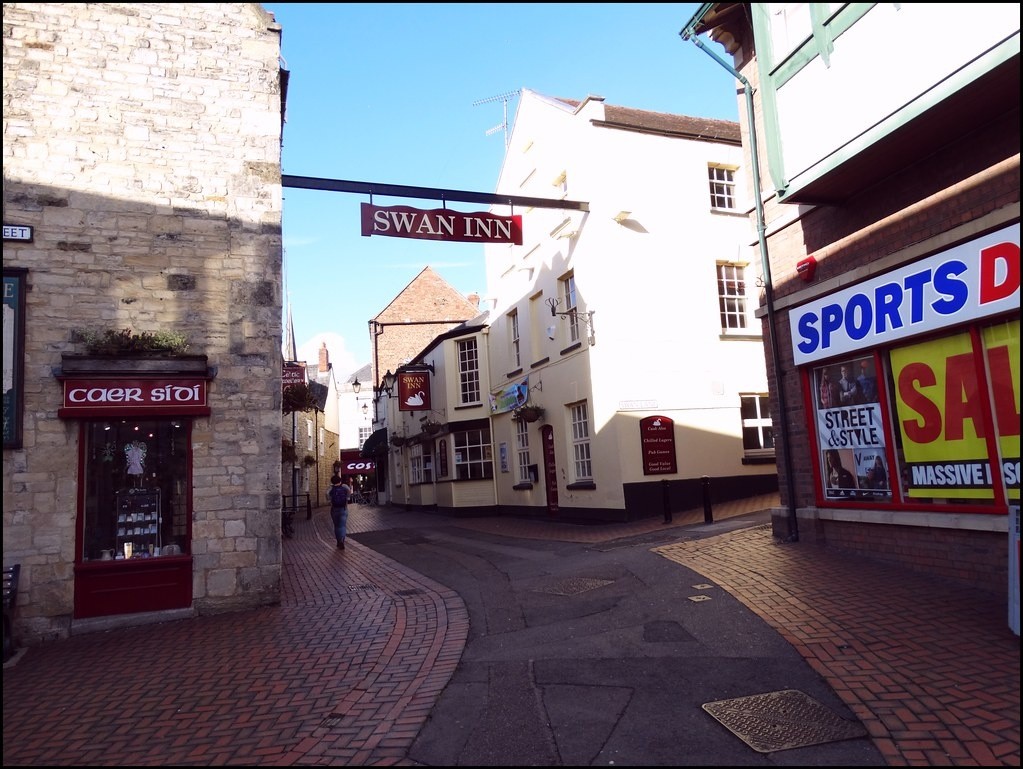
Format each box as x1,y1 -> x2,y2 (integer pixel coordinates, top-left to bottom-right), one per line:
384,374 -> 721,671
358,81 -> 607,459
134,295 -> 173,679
340,541 -> 344,549
337,539 -> 341,546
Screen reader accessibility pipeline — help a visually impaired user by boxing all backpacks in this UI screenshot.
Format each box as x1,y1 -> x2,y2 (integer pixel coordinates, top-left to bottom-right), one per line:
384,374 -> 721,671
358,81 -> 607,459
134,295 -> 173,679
329,483 -> 348,508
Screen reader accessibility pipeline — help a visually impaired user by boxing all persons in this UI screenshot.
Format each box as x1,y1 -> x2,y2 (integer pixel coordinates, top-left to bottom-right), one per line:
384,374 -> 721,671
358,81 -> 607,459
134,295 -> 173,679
328,475 -> 351,550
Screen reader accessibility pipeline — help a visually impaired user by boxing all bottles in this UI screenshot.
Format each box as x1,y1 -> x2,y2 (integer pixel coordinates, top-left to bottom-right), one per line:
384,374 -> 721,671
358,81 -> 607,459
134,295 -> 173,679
100,549 -> 115,561
